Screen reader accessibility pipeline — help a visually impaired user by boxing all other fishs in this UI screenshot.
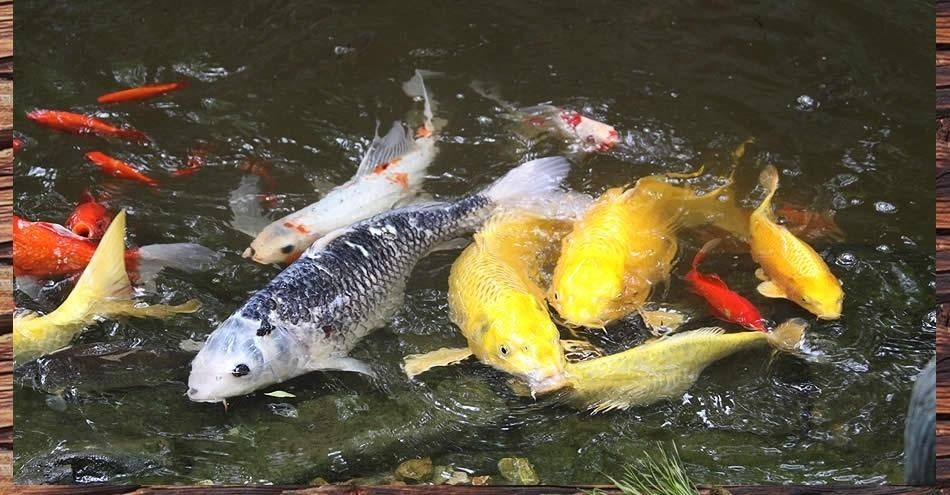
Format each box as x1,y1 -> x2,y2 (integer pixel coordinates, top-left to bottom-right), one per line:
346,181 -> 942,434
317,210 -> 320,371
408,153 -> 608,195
12,65 -> 847,418
